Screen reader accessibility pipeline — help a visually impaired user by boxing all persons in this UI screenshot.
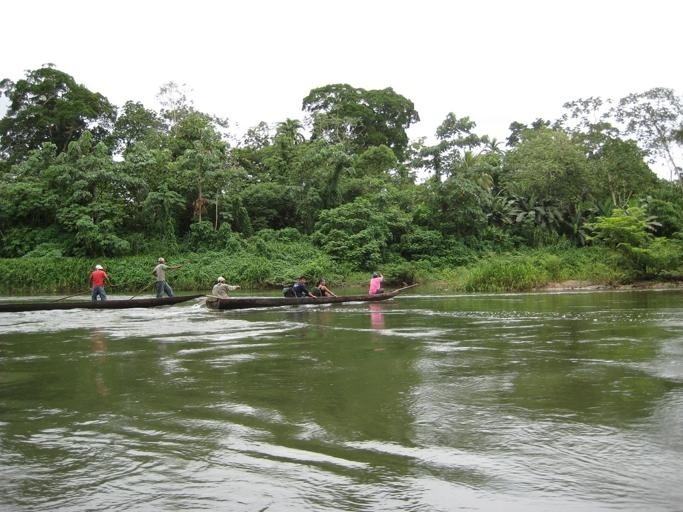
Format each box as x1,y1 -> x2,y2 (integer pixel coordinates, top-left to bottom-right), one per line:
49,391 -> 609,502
369,272 -> 384,294
90,265 -> 114,302
293,276 -> 317,299
151,258 -> 182,299
212,276 -> 240,298
317,279 -> 337,298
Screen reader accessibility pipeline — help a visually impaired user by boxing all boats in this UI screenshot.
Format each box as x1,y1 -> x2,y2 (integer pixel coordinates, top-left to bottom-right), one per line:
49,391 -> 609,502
0,292 -> 207,313
204,281 -> 421,311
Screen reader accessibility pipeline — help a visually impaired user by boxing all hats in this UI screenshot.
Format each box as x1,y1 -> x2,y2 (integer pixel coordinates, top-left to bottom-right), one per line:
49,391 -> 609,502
157,257 -> 165,262
95,264 -> 103,270
217,276 -> 225,282
